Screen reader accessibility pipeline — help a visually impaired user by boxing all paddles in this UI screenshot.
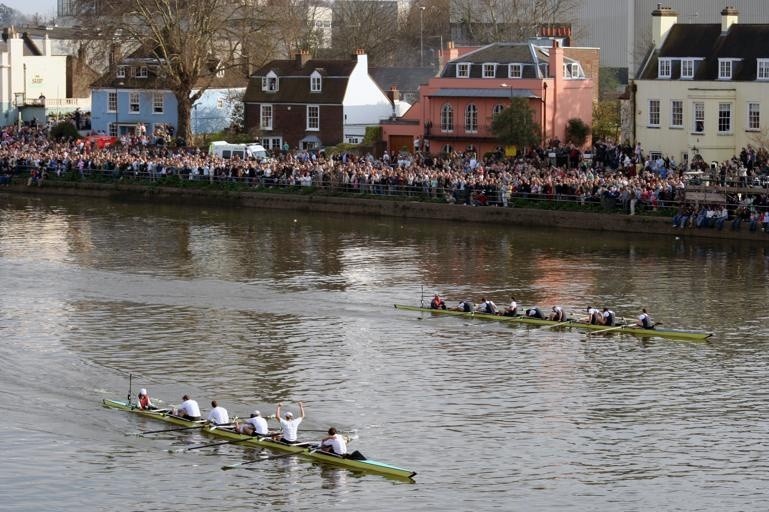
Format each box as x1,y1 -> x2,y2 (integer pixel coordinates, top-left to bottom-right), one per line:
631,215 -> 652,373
416,307 -> 664,340
123,416 -> 349,470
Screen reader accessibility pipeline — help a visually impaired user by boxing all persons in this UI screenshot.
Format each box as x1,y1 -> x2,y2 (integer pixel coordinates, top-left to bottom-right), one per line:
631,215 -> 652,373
234,422 -> 252,433
172,394 -> 202,421
2,120 -> 768,236
243,409 -> 269,436
136,388 -> 153,411
321,427 -> 347,457
637,308 -> 653,329
586,306 -> 602,325
430,293 -> 567,322
597,307 -> 616,326
206,400 -> 231,426
275,402 -> 304,444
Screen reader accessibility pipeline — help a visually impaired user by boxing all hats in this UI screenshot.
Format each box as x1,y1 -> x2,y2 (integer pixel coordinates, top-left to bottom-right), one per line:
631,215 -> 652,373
251,410 -> 260,416
140,389 -> 147,395
283,412 -> 293,419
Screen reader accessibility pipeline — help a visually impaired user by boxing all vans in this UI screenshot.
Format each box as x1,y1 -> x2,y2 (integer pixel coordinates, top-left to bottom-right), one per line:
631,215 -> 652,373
207,141 -> 268,160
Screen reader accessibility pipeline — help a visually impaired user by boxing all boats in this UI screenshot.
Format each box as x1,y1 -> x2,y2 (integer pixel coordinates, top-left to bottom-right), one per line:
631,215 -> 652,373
100,394 -> 420,480
393,301 -> 715,343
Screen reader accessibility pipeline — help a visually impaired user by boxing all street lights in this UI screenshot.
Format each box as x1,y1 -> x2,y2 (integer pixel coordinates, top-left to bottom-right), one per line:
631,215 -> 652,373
113,81 -> 124,138
498,83 -> 514,138
